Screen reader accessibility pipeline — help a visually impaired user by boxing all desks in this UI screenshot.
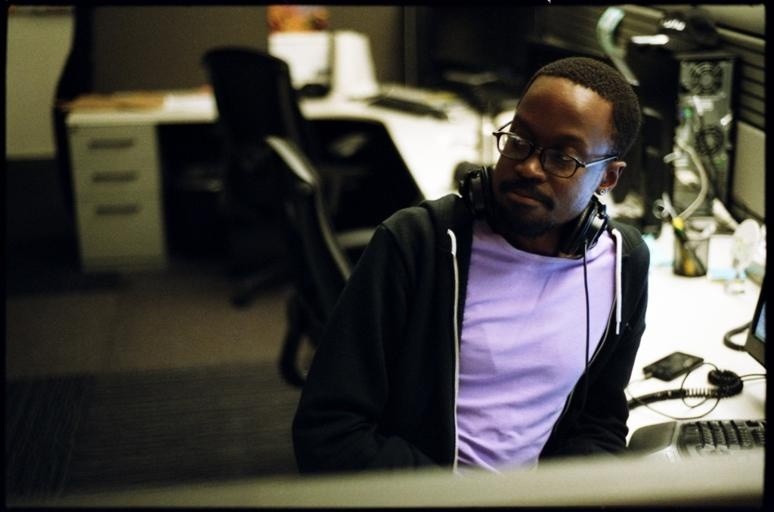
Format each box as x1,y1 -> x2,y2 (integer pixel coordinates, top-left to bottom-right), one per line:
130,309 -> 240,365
64,85 -> 767,449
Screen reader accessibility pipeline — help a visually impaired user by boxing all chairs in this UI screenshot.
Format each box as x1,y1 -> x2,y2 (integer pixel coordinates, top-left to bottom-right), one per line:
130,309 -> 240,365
264,135 -> 378,391
201,48 -> 361,309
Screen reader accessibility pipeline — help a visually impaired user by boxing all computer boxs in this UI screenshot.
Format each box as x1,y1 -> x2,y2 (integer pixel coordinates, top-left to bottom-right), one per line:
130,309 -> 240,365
627,32 -> 743,217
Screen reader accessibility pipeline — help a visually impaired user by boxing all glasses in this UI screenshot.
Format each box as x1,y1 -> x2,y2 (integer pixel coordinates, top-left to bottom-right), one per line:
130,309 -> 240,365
491,120 -> 618,181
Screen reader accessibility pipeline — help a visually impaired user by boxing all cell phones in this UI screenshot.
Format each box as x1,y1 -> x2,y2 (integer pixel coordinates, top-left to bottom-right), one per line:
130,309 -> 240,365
644,352 -> 703,381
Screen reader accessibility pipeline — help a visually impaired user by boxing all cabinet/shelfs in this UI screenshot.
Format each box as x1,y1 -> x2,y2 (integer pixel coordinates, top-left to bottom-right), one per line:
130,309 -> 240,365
71,124 -> 166,274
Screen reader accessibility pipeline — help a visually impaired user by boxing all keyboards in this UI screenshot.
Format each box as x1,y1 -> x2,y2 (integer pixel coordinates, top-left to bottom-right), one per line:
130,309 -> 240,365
628,419 -> 765,452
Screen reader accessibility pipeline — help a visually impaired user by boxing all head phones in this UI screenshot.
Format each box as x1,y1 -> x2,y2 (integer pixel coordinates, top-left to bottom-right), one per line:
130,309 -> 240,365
458,165 -> 608,256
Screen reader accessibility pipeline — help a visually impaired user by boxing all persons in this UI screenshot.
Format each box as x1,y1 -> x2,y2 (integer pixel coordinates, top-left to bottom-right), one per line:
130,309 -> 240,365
291,56 -> 650,478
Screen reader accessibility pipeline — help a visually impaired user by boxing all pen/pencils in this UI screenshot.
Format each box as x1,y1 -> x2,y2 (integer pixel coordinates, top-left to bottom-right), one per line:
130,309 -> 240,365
657,191 -> 706,276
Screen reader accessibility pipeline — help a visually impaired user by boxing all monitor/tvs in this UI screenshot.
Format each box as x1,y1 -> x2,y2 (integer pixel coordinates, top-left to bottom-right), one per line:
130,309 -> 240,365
745,274 -> 767,369
